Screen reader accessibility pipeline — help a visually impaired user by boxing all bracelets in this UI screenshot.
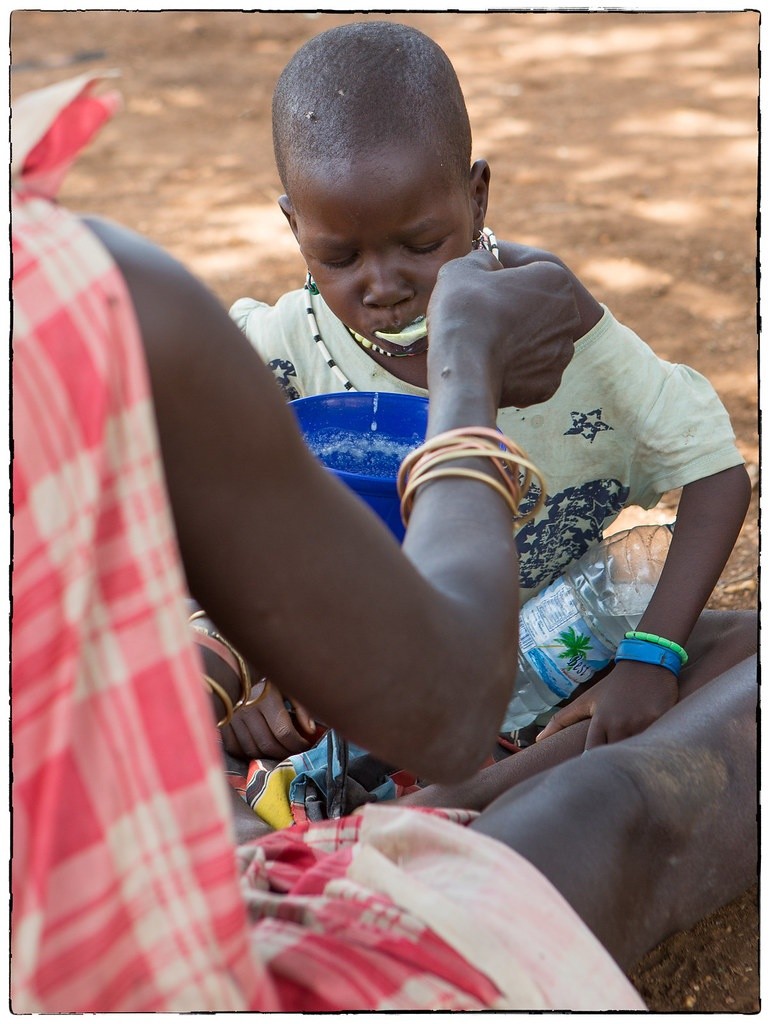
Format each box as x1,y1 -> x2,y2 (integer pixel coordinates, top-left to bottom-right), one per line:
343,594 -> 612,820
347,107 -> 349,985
394,426 -> 547,530
179,608 -> 271,731
614,630 -> 689,678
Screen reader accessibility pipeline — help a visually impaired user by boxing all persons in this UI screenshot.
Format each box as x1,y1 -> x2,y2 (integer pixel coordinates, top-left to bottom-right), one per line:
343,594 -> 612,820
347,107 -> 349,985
218,24 -> 753,815
11,67 -> 757,1016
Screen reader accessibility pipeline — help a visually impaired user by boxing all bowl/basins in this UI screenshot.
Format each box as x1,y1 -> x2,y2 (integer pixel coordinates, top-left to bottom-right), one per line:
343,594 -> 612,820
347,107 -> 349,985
286,392 -> 507,545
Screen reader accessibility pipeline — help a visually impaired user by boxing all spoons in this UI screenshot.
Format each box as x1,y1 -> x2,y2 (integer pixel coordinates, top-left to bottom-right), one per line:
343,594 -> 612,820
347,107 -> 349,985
375,318 -> 427,347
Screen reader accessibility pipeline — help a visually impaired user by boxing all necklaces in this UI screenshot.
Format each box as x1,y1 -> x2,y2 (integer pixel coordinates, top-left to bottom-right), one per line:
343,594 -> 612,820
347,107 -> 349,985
301,227 -> 499,392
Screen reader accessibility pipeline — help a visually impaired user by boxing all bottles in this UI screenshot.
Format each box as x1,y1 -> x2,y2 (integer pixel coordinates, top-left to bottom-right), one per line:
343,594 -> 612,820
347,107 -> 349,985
496,517 -> 680,727
